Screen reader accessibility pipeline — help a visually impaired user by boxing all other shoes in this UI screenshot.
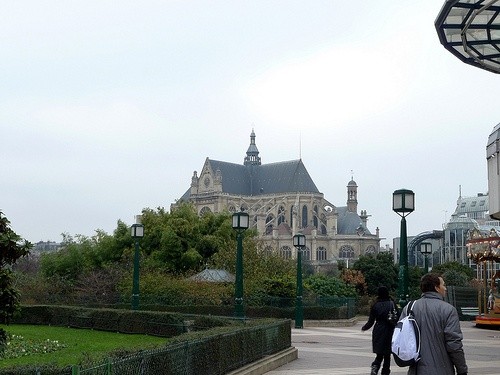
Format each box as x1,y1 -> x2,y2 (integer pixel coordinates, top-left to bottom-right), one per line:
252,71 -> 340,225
371,366 -> 379,375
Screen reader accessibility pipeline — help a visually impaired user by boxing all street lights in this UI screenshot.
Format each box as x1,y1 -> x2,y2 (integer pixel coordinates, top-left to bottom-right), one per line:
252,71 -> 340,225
231,211 -> 250,319
392,188 -> 416,311
130,223 -> 145,309
420,242 -> 433,273
291,233 -> 307,328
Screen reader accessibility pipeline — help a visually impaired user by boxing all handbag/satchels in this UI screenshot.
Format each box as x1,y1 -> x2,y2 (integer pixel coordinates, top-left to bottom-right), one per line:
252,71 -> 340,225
388,301 -> 400,320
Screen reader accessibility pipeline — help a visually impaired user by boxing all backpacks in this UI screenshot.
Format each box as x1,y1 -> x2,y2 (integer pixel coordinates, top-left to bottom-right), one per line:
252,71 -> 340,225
392,300 -> 422,367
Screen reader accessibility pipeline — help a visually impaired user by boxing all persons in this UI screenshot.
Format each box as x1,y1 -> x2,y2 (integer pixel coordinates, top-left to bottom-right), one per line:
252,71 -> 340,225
361,286 -> 401,375
399,273 -> 468,375
